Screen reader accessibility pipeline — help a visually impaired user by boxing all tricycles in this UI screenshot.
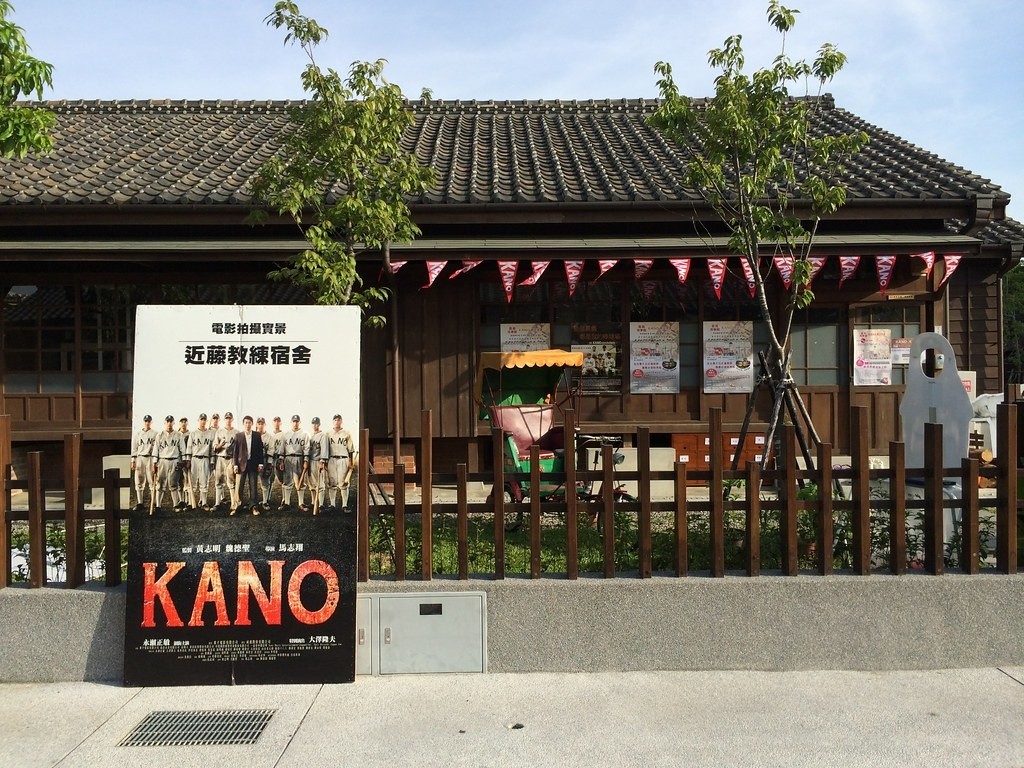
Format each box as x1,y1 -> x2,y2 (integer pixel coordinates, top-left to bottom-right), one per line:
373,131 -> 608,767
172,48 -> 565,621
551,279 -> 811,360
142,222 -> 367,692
471,348 -> 642,555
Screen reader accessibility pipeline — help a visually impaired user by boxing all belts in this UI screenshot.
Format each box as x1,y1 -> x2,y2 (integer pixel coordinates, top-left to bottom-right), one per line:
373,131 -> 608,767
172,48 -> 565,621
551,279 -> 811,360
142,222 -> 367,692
262,453 -> 348,462
138,454 -> 232,463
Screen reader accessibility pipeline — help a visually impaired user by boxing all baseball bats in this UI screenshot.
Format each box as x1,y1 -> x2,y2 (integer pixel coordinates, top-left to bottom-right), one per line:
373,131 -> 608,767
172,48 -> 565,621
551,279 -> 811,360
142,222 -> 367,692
148,473 -> 159,516
312,470 -> 320,515
187,469 -> 197,509
297,468 -> 306,490
343,451 -> 359,484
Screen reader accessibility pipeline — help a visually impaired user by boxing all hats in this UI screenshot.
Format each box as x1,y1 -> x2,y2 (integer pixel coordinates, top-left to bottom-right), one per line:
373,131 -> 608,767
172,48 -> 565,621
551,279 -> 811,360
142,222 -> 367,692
212,413 -> 219,418
224,412 -> 232,419
199,413 -> 207,420
273,417 -> 281,422
165,415 -> 174,421
179,416 -> 187,422
332,414 -> 342,420
311,417 -> 320,424
257,417 -> 265,423
291,415 -> 300,422
143,415 -> 152,421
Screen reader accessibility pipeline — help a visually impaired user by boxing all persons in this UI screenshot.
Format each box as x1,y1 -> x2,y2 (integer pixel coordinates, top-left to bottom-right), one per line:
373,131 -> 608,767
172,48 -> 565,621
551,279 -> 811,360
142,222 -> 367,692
131,411 -> 356,517
581,345 -> 615,377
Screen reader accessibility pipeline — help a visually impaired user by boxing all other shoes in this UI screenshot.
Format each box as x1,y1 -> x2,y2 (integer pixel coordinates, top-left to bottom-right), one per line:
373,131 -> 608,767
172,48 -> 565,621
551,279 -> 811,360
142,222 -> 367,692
132,501 -> 352,516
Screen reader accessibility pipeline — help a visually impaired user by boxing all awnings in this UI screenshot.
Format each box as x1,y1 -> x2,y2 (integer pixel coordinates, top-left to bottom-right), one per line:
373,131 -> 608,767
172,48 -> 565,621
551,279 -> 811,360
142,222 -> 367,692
479,349 -> 584,370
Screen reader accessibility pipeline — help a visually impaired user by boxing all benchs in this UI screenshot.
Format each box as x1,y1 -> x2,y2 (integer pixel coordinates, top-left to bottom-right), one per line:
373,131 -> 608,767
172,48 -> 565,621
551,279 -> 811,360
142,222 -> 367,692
486,404 -> 564,461
